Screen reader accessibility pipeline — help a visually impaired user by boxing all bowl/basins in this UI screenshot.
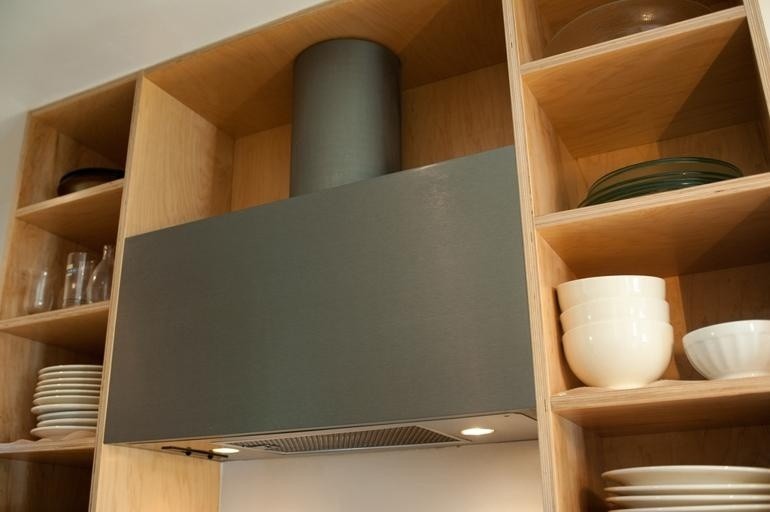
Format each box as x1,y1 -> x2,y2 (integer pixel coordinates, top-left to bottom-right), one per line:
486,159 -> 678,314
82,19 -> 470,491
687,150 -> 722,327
557,273 -> 770,388
543,0 -> 738,57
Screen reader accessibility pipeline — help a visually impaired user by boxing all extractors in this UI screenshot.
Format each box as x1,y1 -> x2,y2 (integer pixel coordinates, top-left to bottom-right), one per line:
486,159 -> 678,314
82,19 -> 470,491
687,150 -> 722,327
103,38 -> 539,461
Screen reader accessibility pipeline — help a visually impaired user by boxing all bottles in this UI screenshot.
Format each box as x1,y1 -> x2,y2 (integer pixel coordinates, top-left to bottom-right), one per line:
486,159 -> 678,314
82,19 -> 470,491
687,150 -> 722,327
86,244 -> 114,305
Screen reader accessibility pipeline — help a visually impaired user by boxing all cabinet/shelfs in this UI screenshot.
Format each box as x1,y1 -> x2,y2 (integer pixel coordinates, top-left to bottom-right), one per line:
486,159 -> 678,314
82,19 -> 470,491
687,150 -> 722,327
0,0 -> 770,512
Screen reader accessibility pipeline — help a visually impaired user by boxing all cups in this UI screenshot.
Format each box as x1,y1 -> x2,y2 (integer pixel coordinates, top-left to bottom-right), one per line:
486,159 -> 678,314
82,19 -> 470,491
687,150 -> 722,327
21,245 -> 95,315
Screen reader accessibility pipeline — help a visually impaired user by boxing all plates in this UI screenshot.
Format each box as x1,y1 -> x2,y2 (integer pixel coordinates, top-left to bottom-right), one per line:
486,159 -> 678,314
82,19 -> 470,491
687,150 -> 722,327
601,465 -> 770,512
575,155 -> 745,209
31,360 -> 101,441
